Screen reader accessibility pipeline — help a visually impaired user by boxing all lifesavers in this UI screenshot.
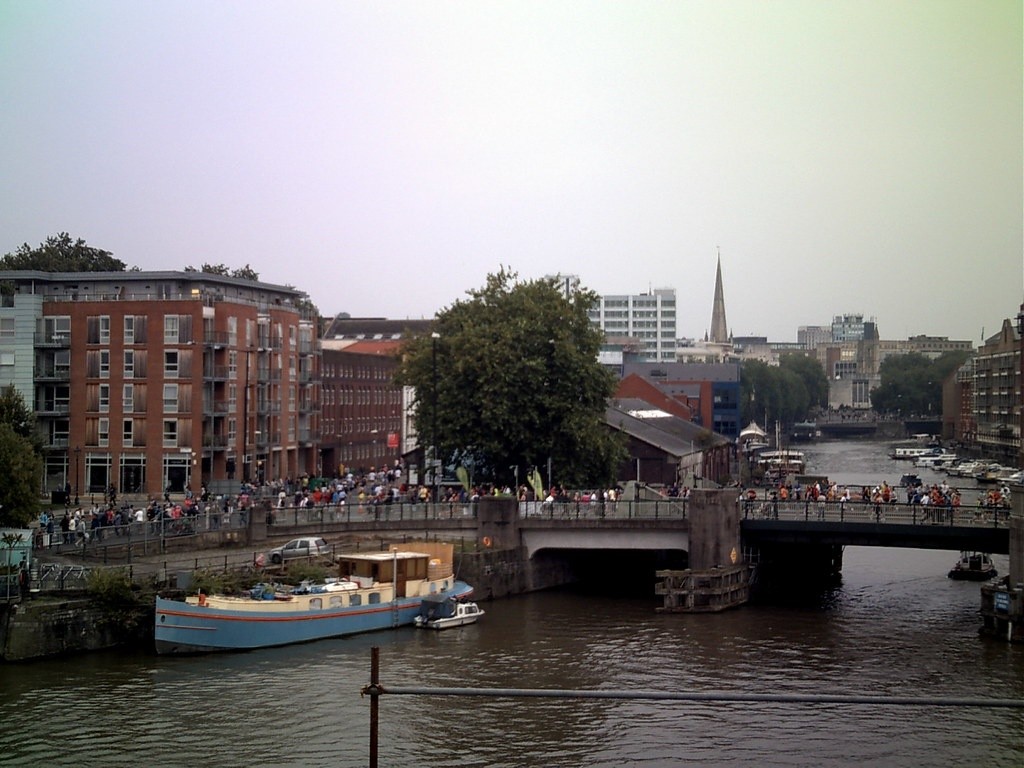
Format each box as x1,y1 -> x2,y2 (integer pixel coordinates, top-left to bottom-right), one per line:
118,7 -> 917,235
483,536 -> 490,546
199,594 -> 206,603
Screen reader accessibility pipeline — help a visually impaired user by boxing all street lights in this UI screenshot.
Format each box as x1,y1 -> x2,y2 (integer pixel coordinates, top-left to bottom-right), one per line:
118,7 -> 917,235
432,332 -> 441,461
190,341 -> 220,493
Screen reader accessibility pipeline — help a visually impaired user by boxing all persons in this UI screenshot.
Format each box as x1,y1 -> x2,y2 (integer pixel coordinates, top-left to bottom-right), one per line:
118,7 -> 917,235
805,479 -> 854,522
973,482 -> 1012,527
906,480 -> 961,525
730,471 -> 802,521
358,459 -> 432,520
266,465 -> 358,524
859,480 -> 899,523
542,484 -> 623,519
442,484 -> 529,516
657,485 -> 691,509
40,480 -> 146,548
147,478 -> 264,537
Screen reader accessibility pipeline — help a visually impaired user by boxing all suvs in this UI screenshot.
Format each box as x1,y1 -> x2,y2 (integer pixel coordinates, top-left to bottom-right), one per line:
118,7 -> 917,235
268,537 -> 330,564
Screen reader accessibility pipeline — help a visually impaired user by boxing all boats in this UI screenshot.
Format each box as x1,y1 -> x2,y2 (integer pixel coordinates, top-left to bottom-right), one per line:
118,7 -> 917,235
997,472 -> 1024,493
889,446 -> 945,459
948,549 -> 997,580
913,453 -> 999,478
154,543 -> 474,654
738,417 -> 831,486
413,596 -> 486,630
976,468 -> 1019,483
